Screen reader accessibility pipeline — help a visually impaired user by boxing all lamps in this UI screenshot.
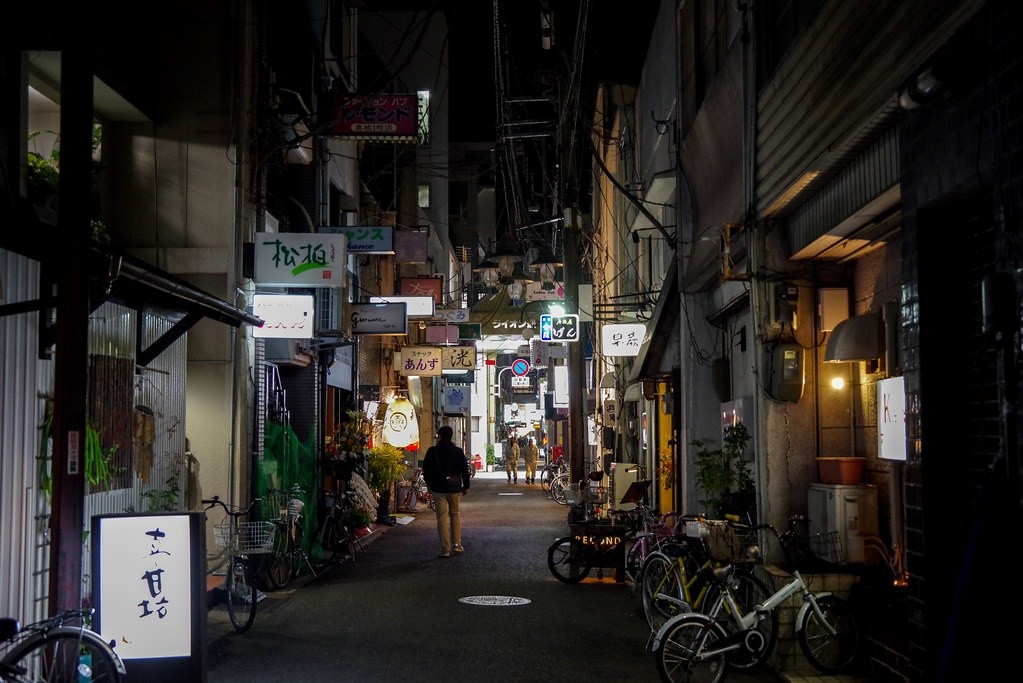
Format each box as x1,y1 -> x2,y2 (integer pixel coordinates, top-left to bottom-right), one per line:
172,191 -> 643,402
529,247 -> 564,292
486,239 -> 527,284
531,271 -> 542,293
633,224 -> 677,243
506,276 -> 522,307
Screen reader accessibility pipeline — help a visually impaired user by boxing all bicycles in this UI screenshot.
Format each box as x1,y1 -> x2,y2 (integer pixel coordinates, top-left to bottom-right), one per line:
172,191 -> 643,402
651,511 -> 865,683
466,456 -> 476,478
198,494 -> 279,634
395,460 -> 437,515
537,446 -> 780,674
267,481 -> 360,589
0,606 -> 127,683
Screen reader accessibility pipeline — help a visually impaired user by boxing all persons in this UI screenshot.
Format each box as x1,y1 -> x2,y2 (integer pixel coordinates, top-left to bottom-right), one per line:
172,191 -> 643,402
423,426 -> 471,557
505,435 -> 539,485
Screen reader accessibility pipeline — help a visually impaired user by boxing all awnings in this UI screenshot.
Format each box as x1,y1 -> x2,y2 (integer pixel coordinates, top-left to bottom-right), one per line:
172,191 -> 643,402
0,205 -> 264,370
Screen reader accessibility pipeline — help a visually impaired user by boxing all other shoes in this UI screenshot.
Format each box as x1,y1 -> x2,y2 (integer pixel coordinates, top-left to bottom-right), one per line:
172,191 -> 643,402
508,478 -> 511,481
440,552 -> 450,556
453,544 -> 465,552
526,480 -> 530,484
514,478 -> 517,484
531,481 -> 535,484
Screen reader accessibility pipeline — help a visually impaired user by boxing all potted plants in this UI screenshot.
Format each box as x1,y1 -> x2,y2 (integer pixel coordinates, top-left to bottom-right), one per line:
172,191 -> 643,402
367,443 -> 407,520
485,443 -> 496,473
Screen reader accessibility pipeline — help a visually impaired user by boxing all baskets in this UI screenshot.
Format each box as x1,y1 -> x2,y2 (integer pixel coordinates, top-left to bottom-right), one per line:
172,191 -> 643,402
564,482 -> 583,506
718,528 -> 770,567
806,531 -> 845,564
329,502 -> 353,518
214,522 -> 276,555
269,494 -> 304,521
589,485 -> 609,503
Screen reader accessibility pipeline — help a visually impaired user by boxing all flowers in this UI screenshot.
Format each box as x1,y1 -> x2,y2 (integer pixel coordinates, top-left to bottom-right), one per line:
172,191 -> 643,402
327,409 -> 374,472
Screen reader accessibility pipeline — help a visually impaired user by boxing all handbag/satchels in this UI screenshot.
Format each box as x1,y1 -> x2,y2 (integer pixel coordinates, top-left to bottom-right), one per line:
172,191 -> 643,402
441,474 -> 461,488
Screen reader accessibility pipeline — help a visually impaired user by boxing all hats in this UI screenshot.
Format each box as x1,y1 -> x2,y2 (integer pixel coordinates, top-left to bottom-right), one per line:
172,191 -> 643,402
436,425 -> 453,435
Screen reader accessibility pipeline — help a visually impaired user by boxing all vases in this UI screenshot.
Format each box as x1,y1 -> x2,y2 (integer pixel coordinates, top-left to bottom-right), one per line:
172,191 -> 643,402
814,457 -> 866,484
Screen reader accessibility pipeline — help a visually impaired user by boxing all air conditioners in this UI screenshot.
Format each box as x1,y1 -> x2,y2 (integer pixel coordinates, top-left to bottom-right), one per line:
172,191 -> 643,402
608,463 -> 638,511
319,286 -> 342,331
808,483 -> 879,565
